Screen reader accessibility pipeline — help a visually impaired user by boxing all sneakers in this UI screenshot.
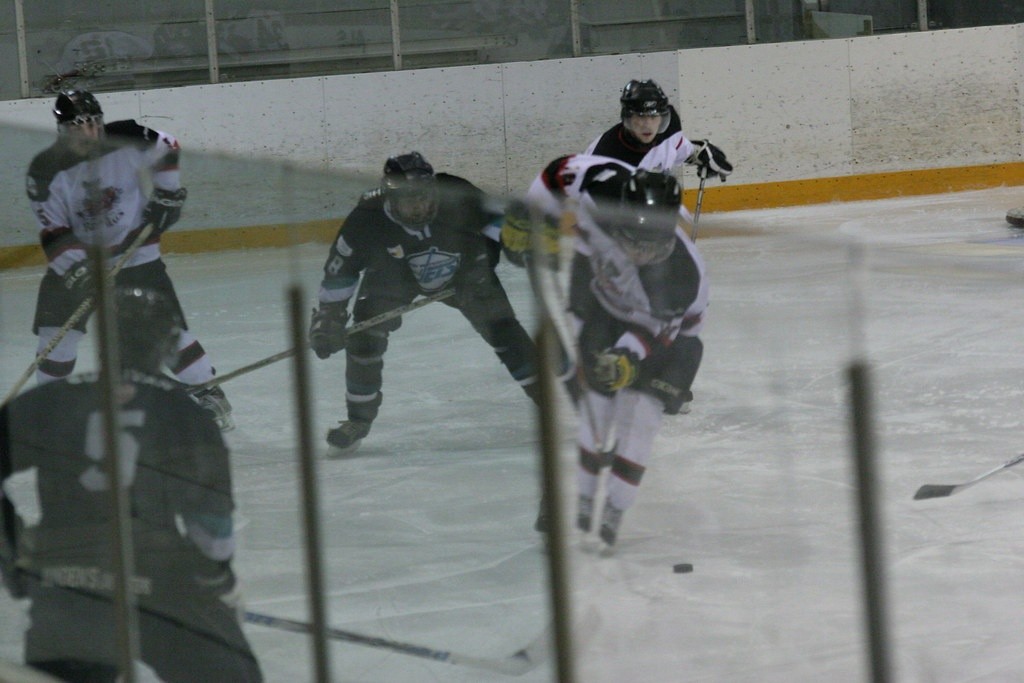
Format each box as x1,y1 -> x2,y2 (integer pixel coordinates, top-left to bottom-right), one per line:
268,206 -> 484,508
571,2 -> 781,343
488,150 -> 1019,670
595,499 -> 623,550
577,493 -> 597,534
324,420 -> 371,459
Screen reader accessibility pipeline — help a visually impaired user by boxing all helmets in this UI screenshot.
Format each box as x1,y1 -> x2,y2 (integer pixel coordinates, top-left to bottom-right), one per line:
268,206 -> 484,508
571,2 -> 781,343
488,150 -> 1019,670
381,152 -> 440,230
53,89 -> 104,158
617,169 -> 681,248
100,287 -> 181,376
618,78 -> 670,118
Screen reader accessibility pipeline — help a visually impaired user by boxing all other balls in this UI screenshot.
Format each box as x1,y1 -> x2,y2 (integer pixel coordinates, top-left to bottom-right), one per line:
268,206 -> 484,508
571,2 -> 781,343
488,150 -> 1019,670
674,563 -> 693,572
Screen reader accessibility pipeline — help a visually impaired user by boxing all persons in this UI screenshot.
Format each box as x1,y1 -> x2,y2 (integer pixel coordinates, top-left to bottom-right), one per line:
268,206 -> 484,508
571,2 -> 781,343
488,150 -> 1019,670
520,152 -> 706,555
25,92 -> 238,432
563,81 -> 729,344
305,150 -> 552,446
0,286 -> 265,683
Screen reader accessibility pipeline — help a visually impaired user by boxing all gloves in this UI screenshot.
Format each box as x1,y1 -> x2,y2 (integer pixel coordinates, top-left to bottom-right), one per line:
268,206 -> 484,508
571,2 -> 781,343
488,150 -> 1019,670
60,258 -> 102,311
501,199 -> 558,268
309,308 -> 351,359
592,346 -> 639,392
696,139 -> 733,182
143,188 -> 186,238
185,538 -> 237,597
455,248 -> 492,293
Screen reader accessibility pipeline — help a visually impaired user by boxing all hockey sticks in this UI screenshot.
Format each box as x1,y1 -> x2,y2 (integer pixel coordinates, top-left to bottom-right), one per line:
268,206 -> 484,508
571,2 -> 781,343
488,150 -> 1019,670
185,286 -> 467,391
912,451 -> 1024,502
1,220 -> 159,404
676,165 -> 708,415
236,601 -> 581,677
